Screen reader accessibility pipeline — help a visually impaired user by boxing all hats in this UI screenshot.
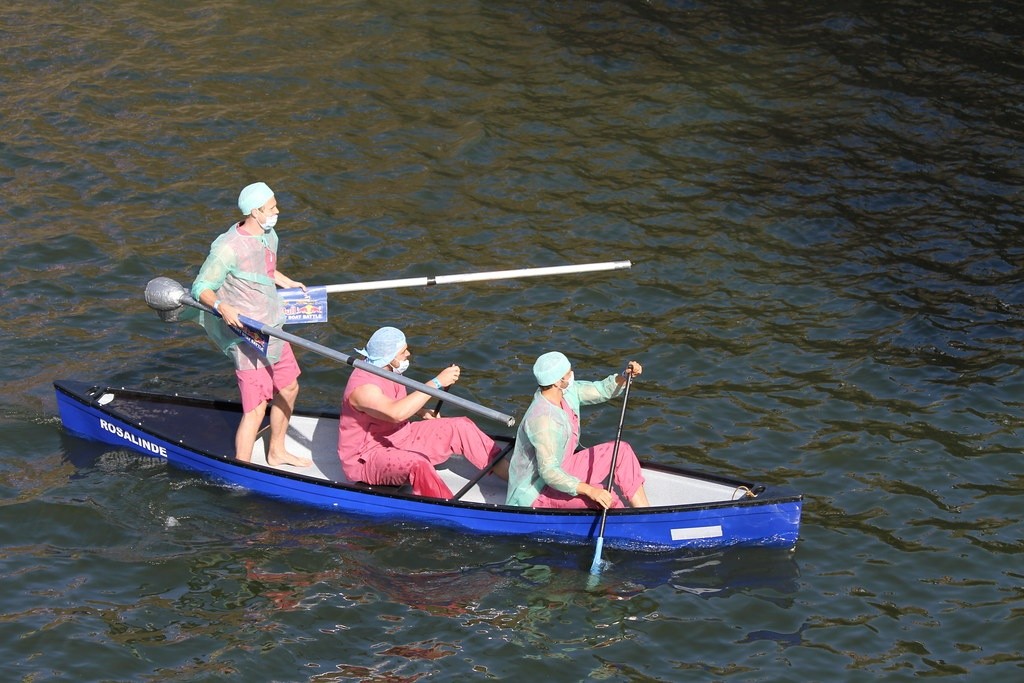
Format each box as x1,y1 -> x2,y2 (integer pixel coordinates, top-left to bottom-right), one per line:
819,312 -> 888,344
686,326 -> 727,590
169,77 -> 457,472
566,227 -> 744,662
367,327 -> 407,369
533,351 -> 571,386
238,182 -> 274,216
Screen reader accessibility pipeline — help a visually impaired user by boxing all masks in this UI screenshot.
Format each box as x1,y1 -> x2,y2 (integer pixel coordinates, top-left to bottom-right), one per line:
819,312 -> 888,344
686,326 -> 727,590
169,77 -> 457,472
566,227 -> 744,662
389,359 -> 409,374
256,209 -> 278,230
559,371 -> 574,395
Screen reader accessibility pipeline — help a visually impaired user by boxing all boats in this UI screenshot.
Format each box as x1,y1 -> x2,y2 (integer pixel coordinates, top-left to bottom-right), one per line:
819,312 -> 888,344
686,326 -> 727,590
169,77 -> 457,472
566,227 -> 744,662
54,379 -> 803,556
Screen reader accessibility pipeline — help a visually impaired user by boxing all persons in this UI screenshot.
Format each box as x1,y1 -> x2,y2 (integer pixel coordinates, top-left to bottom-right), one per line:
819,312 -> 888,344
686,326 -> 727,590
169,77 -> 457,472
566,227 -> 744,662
337,327 -> 510,499
506,351 -> 648,509
185,181 -> 313,467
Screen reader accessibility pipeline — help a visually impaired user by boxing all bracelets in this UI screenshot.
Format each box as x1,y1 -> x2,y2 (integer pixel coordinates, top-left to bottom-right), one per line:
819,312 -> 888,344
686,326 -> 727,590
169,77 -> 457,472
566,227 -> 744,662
213,300 -> 223,310
432,377 -> 441,388
622,371 -> 633,382
